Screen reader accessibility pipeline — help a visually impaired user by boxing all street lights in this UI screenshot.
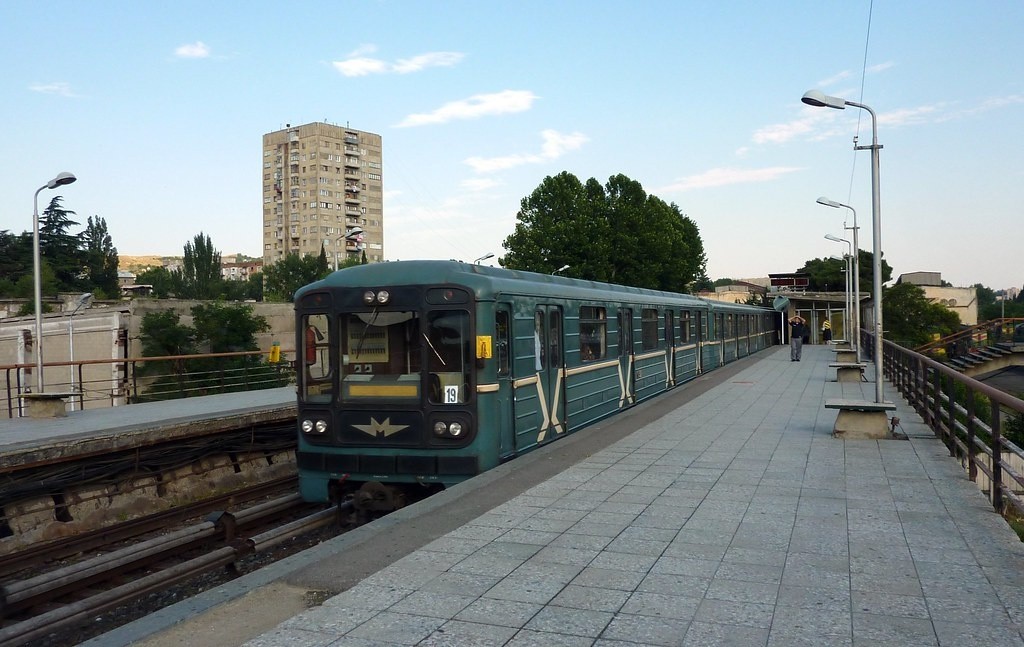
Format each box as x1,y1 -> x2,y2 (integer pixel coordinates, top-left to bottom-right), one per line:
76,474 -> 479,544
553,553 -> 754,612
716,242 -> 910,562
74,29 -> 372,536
551,265 -> 570,276
816,197 -> 861,365
334,227 -> 363,272
825,234 -> 854,351
801,90 -> 889,403
473,253 -> 495,265
830,255 -> 848,341
33,172 -> 76,393
69,293 -> 92,412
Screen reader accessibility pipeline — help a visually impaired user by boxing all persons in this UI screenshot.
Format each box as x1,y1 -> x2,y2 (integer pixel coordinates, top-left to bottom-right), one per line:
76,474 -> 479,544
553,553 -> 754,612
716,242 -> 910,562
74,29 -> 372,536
788,316 -> 806,362
822,317 -> 831,345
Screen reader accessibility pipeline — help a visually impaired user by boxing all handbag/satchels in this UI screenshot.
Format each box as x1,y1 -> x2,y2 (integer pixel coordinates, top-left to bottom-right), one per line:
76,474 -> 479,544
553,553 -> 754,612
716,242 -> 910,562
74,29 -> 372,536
821,327 -> 825,331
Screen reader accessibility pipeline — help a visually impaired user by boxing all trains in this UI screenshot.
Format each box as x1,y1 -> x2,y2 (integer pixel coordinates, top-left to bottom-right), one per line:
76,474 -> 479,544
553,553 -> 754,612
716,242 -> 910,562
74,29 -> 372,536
294,260 -> 775,503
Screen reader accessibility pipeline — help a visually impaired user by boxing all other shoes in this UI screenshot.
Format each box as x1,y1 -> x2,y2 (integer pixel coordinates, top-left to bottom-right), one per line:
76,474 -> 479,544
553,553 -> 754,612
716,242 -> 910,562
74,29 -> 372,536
796,359 -> 800,361
791,359 -> 796,362
